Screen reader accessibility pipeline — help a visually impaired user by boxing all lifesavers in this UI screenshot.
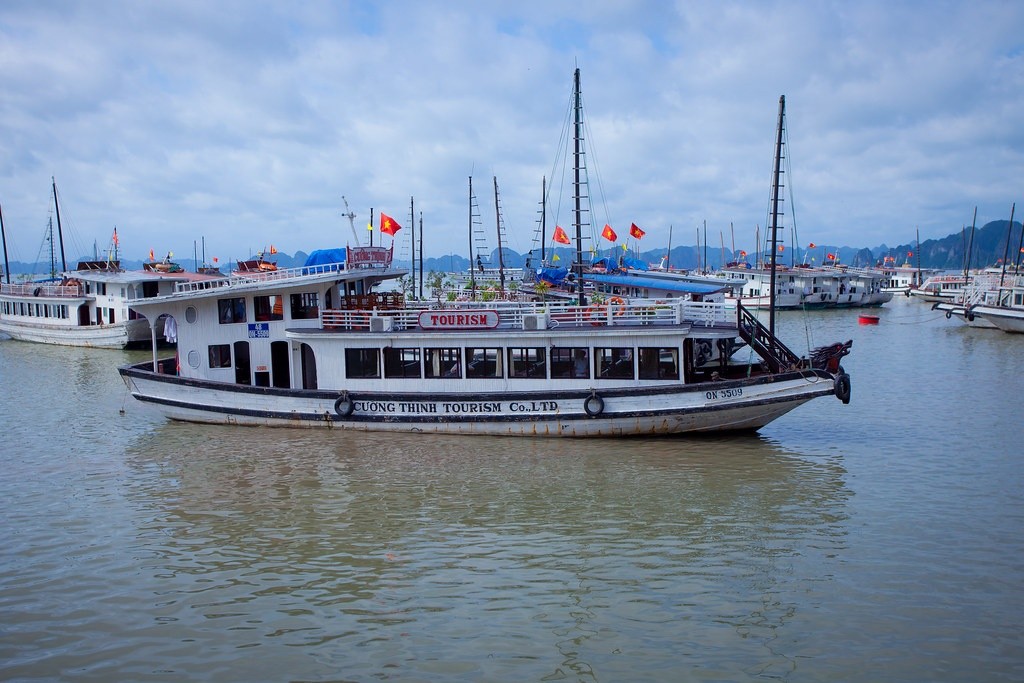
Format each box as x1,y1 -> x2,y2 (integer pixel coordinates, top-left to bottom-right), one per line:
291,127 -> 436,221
583,394 -> 605,417
65,280 -> 82,295
350,309 -> 369,330
586,304 -> 608,327
33,287 -> 40,297
323,308 -> 343,329
334,396 -> 354,418
605,296 -> 625,316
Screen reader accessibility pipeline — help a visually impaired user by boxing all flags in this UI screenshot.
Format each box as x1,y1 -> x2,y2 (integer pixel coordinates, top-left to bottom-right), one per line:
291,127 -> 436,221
740,250 -> 747,256
602,224 -> 618,243
590,246 -> 597,255
621,243 -> 627,250
809,242 -> 815,248
213,257 -> 218,261
149,250 -> 155,261
367,223 -> 373,230
827,253 -> 836,260
168,250 -> 174,260
553,253 -> 560,261
777,243 -> 784,252
270,244 -> 277,254
997,247 -> 1024,263
630,224 -> 645,240
380,212 -> 401,237
553,225 -> 571,245
907,251 -> 913,257
112,229 -> 119,244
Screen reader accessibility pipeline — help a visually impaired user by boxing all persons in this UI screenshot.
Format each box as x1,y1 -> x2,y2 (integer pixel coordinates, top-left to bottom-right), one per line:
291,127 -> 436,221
450,354 -> 473,377
574,350 -> 589,378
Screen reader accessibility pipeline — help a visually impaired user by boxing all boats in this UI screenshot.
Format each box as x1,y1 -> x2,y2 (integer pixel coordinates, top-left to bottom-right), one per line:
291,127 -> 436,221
721,264 -> 894,309
128,239 -> 224,282
971,203 -> 1024,333
229,245 -> 301,283
932,264 -> 1018,329
873,259 -> 941,294
115,68 -> 856,437
910,274 -> 974,302
455,267 -> 526,283
849,264 -> 946,295
0,176 -> 195,349
517,266 -> 759,322
589,256 -> 748,311
